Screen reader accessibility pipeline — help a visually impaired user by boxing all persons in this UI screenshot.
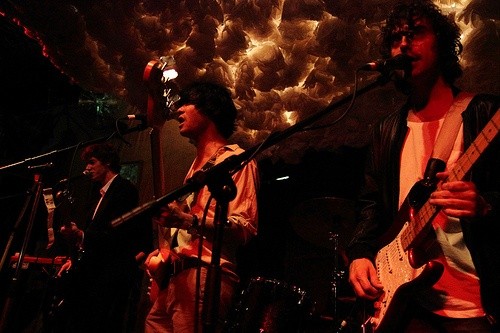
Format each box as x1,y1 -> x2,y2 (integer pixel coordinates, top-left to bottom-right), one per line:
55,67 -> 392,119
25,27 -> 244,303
56,144 -> 153,333
144,80 -> 258,333
348,3 -> 500,333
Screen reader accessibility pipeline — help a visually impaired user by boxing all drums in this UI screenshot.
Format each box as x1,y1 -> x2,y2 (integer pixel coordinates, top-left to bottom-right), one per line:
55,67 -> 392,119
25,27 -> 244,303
220,276 -> 318,333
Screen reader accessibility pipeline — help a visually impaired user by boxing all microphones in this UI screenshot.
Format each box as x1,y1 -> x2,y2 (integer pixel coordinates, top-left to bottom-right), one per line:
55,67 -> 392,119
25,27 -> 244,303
362,54 -> 412,72
134,107 -> 177,120
30,162 -> 55,169
61,170 -> 90,183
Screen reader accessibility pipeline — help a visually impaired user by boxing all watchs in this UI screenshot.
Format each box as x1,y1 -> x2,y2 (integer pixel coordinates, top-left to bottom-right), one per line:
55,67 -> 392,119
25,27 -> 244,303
187,215 -> 198,234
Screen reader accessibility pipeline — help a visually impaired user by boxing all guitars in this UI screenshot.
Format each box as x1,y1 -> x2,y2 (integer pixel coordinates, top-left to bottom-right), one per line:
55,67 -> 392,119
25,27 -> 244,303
142,60 -> 180,304
363,105 -> 499,332
